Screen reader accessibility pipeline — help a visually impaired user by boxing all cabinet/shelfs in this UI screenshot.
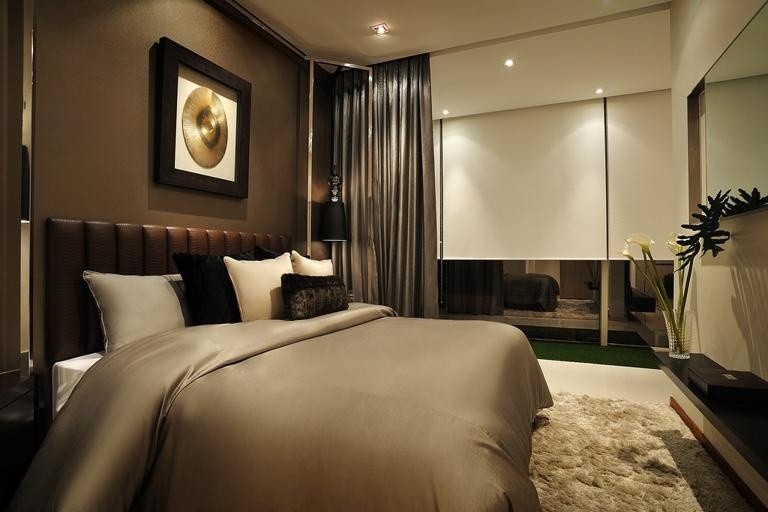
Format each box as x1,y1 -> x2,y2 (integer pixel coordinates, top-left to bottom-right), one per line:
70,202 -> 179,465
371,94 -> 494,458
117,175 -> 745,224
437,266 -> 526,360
653,354 -> 768,483
629,288 -> 656,313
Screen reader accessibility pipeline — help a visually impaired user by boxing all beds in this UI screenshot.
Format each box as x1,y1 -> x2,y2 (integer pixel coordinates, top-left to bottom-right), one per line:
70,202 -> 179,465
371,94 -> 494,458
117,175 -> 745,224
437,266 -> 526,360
9,219 -> 553,512
503,272 -> 559,311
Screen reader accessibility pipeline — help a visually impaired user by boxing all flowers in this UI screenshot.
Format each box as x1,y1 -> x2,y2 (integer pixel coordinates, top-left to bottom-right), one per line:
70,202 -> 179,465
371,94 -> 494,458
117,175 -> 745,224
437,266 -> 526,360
619,189 -> 732,355
724,189 -> 768,215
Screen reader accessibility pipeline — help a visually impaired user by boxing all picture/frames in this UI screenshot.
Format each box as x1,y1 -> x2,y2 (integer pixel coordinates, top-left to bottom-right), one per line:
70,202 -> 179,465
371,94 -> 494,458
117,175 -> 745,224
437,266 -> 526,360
154,36 -> 251,199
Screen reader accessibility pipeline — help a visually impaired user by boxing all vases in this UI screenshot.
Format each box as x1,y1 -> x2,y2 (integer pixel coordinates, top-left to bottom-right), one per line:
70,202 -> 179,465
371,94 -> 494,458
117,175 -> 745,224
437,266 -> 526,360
662,309 -> 694,359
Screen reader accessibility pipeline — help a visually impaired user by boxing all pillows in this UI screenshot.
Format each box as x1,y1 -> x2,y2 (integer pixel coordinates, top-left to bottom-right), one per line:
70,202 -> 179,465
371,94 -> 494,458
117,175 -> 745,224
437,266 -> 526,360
173,246 -> 260,325
292,250 -> 333,277
281,273 -> 347,321
83,269 -> 192,354
254,245 -> 280,259
223,253 -> 295,323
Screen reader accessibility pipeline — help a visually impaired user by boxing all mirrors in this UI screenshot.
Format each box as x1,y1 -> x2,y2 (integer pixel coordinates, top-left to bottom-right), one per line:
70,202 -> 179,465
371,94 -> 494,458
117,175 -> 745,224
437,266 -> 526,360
705,0 -> 768,220
437,260 -> 603,344
608,261 -> 672,348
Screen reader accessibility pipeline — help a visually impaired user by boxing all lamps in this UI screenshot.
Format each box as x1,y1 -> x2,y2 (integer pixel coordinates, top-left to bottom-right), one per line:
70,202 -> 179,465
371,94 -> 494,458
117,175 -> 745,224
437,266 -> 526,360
371,24 -> 390,39
320,167 -> 348,242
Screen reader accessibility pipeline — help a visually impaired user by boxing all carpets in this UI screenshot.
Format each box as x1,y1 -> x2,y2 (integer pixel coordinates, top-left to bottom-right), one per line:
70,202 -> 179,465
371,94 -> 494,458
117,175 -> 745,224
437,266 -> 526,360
503,299 -> 600,319
529,392 -> 759,512
529,340 -> 663,370
